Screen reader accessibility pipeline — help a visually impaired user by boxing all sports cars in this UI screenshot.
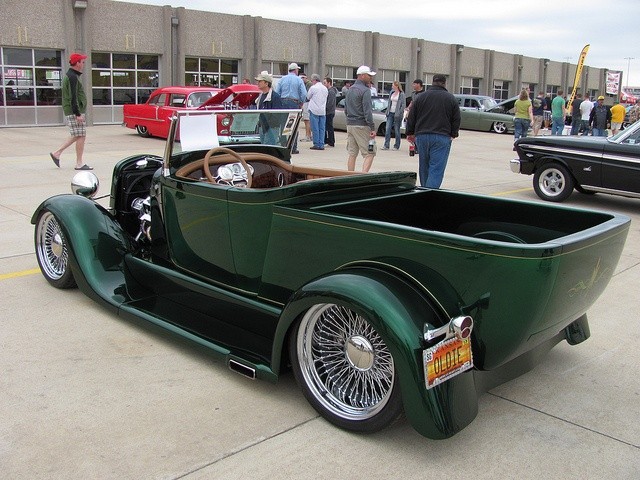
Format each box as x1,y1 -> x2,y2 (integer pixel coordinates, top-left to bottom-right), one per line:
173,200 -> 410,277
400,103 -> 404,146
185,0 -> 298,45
333,93 -> 410,137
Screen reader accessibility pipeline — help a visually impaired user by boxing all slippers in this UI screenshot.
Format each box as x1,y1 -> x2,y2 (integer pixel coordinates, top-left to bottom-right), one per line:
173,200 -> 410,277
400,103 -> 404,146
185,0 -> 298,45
50,152 -> 60,168
74,164 -> 93,170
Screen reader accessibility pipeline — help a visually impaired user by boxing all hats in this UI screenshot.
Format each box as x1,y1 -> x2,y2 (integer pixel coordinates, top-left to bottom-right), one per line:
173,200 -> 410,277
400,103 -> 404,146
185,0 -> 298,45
288,63 -> 301,70
69,53 -> 88,65
598,96 -> 604,100
258,71 -> 272,76
356,65 -> 376,75
254,75 -> 272,83
433,75 -> 446,82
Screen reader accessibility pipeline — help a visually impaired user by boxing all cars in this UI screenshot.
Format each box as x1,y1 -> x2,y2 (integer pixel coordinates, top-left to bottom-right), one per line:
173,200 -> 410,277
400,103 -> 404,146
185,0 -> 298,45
122,86 -> 263,144
31,108 -> 632,440
452,93 -> 520,134
510,118 -> 640,202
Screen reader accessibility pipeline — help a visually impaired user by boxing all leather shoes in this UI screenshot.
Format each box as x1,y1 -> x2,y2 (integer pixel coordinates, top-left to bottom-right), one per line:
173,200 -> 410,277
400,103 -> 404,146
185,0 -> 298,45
310,146 -> 319,150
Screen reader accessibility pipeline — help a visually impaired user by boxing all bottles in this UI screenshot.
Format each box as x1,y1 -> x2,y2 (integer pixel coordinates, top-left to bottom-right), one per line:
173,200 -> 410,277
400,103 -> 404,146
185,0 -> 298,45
369,133 -> 374,152
409,136 -> 415,157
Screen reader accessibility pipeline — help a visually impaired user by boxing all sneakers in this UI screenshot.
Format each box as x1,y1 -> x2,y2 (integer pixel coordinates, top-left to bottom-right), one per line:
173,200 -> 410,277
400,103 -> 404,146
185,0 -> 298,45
300,138 -> 311,142
380,147 -> 389,150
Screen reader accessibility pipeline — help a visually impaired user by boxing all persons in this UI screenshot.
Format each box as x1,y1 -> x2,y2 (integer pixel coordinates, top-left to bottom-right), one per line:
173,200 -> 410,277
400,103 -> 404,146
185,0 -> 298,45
5,78 -> 54,101
366,81 -> 378,97
323,78 -> 337,147
570,93 -> 584,135
404,79 -> 425,154
345,66 -> 377,173
342,81 -> 354,96
243,79 -> 250,84
50,54 -> 94,170
588,95 -> 612,136
275,63 -> 307,154
380,81 -> 406,150
514,90 -> 534,142
610,100 -> 625,136
405,74 -> 461,189
306,74 -> 328,150
543,93 -> 552,130
251,70 -> 283,145
551,90 -> 566,135
579,93 -> 595,135
532,92 -> 547,136
